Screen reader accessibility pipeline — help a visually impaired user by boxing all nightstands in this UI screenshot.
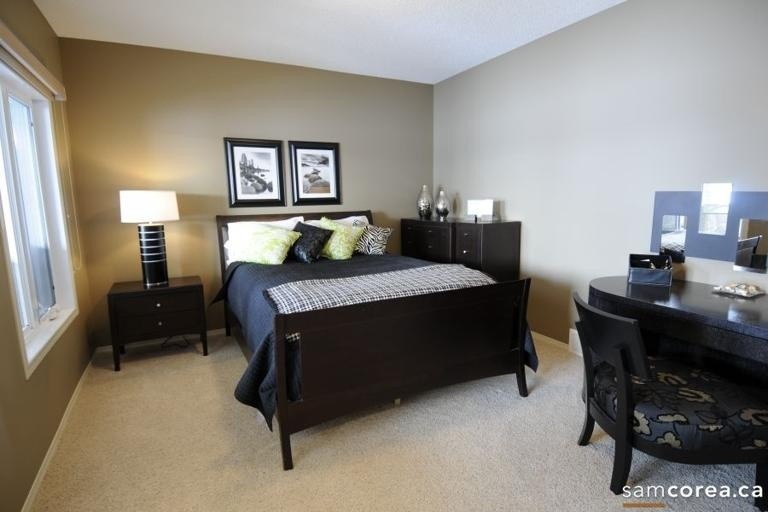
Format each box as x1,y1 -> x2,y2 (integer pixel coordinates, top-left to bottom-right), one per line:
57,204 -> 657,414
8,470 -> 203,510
106,273 -> 210,372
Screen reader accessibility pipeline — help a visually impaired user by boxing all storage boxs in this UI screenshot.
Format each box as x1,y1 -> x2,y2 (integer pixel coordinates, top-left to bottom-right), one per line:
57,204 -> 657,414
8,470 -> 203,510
627,252 -> 674,289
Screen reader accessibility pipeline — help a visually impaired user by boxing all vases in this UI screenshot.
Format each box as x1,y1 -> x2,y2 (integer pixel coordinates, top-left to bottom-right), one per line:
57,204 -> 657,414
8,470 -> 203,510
433,190 -> 452,223
416,184 -> 435,219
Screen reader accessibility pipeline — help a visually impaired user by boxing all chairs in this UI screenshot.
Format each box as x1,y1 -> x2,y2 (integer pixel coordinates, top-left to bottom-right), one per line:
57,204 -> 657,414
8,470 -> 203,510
569,290 -> 767,512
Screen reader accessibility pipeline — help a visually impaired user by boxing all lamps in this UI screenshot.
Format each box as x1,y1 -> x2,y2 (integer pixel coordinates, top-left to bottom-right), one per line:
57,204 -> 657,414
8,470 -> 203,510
117,187 -> 182,290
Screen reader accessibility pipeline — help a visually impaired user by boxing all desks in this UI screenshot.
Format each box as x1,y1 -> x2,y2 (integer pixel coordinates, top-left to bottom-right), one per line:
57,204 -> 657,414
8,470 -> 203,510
578,273 -> 767,408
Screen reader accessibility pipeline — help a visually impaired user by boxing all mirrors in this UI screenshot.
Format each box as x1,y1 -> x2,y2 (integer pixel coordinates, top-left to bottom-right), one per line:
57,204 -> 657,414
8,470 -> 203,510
696,181 -> 733,237
732,217 -> 768,274
659,214 -> 688,263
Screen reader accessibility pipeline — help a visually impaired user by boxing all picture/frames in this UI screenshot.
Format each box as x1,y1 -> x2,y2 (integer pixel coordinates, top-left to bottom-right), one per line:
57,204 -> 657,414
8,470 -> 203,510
222,135 -> 286,209
287,139 -> 341,207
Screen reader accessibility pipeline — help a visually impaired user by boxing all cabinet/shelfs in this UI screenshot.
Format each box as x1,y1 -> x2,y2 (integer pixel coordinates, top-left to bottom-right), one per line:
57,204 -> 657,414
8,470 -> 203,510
400,216 -> 522,281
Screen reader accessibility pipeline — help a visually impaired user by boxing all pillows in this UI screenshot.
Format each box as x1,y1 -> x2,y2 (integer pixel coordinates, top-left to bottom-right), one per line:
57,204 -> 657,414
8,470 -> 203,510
222,214 -> 396,267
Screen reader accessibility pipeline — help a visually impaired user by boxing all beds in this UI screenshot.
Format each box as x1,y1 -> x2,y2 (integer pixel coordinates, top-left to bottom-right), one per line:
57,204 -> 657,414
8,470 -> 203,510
215,209 -> 539,470
